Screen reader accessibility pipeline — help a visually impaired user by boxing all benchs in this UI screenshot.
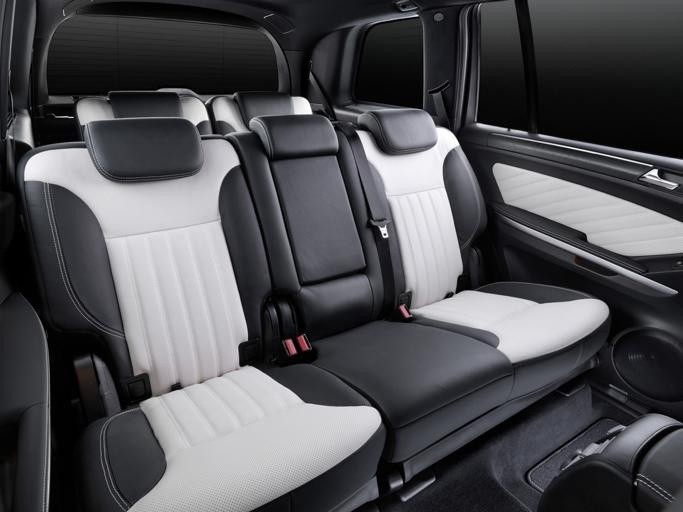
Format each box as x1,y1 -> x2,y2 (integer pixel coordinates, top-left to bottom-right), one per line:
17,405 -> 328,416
0,90 -> 610,512
